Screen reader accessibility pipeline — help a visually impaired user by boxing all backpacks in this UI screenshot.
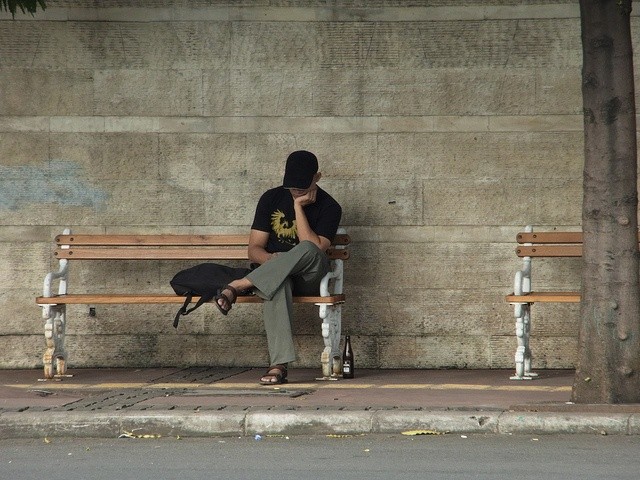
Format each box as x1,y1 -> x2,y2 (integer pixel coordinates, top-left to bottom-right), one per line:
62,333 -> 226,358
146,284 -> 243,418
169,263 -> 252,328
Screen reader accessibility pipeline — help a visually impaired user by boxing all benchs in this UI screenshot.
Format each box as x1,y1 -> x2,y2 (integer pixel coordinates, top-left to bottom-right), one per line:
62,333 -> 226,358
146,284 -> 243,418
35,229 -> 351,381
507,227 -> 584,380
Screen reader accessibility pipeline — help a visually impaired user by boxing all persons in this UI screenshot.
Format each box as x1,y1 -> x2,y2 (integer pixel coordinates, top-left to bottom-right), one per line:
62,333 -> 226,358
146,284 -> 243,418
214,149 -> 343,387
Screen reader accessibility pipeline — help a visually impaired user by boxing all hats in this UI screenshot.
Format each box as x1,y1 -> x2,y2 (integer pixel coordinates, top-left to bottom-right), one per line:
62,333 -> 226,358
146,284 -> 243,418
282,150 -> 318,189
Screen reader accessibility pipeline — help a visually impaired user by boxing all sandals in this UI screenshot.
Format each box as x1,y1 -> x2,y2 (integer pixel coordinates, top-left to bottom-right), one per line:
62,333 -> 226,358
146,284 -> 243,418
215,285 -> 237,316
258,364 -> 288,384
